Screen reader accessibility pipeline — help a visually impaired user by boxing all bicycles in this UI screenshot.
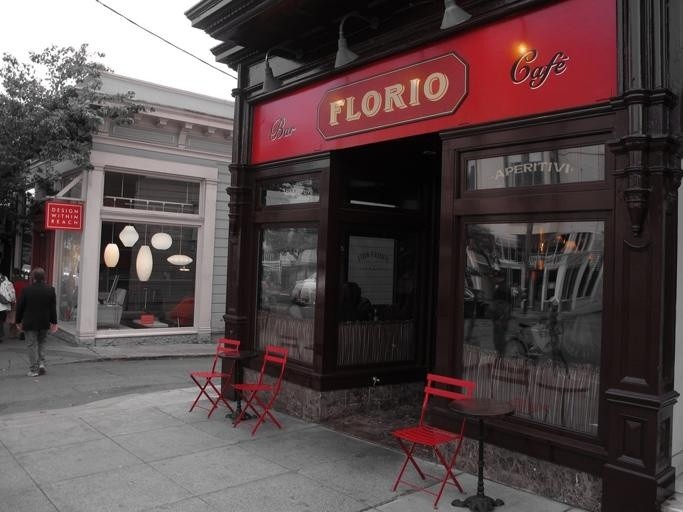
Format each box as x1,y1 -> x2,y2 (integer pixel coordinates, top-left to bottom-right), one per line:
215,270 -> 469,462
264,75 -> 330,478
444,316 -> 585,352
501,320 -> 569,380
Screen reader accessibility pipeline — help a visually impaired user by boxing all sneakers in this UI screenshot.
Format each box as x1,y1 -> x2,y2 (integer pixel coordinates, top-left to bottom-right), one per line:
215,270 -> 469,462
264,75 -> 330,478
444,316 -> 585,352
28,371 -> 39,377
39,360 -> 48,375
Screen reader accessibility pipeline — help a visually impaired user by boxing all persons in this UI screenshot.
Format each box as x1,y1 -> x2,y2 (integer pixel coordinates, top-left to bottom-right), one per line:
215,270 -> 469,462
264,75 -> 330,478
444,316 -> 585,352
490,270 -> 514,362
340,281 -> 375,321
0,271 -> 18,343
12,271 -> 29,341
15,267 -> 58,377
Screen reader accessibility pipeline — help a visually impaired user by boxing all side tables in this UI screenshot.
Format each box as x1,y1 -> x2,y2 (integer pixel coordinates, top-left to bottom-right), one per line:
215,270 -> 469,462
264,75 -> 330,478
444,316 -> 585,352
448,397 -> 516,512
538,377 -> 589,426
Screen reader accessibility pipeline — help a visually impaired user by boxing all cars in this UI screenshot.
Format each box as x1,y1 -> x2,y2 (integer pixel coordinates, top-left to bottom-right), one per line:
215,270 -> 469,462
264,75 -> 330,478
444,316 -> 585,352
465,287 -> 511,319
291,271 -> 317,304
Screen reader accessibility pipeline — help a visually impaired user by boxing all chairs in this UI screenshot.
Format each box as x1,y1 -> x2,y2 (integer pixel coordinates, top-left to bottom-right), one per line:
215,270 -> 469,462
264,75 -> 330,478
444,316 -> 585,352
190,338 -> 287,436
389,373 -> 475,507
488,365 -> 552,424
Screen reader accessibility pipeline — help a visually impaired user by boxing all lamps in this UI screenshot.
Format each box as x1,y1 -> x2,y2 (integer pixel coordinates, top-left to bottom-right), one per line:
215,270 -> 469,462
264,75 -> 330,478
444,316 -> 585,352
333,14 -> 386,68
439,1 -> 473,29
262,44 -> 304,95
103,195 -> 195,282
